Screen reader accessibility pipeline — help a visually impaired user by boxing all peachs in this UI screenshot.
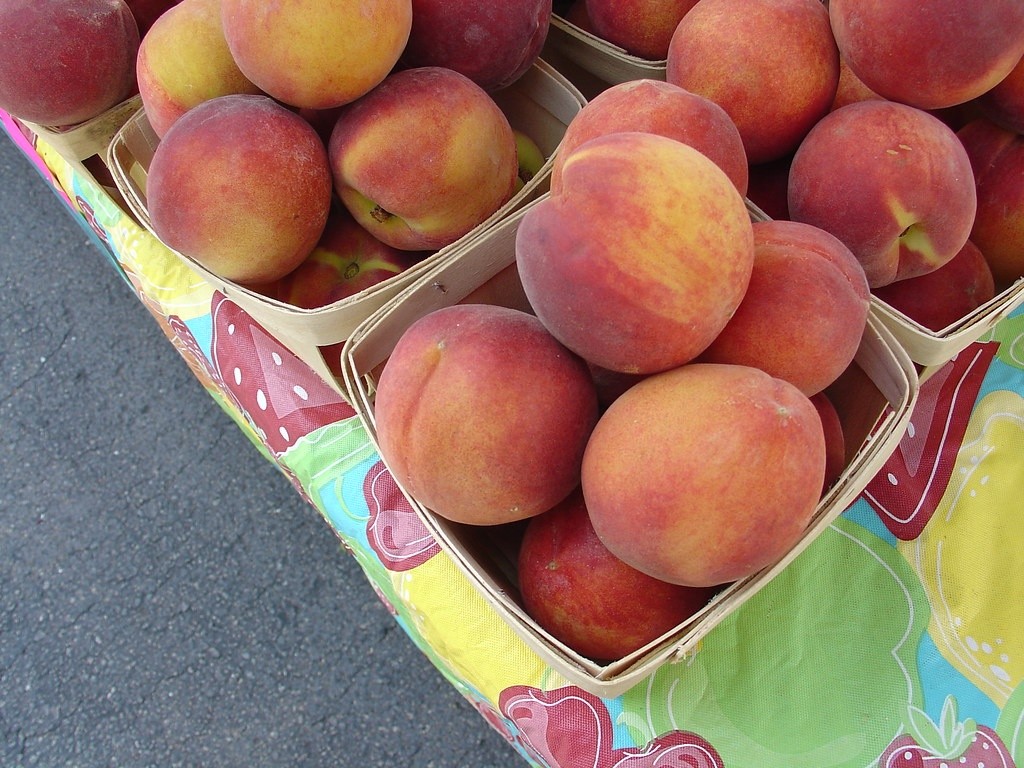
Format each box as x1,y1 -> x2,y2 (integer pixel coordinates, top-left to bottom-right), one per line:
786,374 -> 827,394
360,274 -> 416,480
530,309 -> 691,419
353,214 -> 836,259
0,0 -> 1024,665
